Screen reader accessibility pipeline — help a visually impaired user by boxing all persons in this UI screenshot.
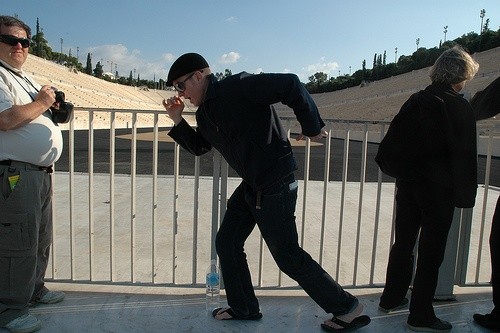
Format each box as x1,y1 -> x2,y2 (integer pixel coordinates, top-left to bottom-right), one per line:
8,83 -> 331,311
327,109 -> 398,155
369,49 -> 500,333
162,52 -> 371,333
0,15 -> 68,333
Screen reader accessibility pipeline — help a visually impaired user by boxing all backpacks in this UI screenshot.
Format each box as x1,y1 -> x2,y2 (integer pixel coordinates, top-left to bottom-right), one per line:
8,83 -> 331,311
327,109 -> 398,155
376,90 -> 464,182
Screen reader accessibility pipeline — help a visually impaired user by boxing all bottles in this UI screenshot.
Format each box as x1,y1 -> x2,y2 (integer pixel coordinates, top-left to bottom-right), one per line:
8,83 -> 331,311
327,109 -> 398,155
206,264 -> 220,311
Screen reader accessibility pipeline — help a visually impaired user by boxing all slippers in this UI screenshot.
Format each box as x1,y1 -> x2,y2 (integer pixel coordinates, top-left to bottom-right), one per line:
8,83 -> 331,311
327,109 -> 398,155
213,308 -> 262,320
321,315 -> 371,332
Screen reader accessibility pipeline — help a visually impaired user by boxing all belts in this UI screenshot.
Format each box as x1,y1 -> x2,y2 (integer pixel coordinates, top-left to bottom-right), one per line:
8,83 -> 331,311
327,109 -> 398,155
0,160 -> 53,173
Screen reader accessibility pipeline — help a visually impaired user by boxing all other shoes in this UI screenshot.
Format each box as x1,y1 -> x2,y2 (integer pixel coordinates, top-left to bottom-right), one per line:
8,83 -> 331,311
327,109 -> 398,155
379,298 -> 409,312
36,291 -> 65,303
473,314 -> 500,333
407,314 -> 452,332
4,313 -> 40,333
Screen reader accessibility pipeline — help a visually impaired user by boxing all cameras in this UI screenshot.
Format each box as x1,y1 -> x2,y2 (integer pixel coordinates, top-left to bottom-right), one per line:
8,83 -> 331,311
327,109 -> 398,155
49,87 -> 65,104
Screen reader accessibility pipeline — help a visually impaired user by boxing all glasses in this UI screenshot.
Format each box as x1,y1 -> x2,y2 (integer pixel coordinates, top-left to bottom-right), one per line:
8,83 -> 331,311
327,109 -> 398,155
172,71 -> 204,92
0,35 -> 30,48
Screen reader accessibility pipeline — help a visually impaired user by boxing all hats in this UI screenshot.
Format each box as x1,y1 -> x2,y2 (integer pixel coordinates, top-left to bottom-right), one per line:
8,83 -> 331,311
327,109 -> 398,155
166,53 -> 209,86
